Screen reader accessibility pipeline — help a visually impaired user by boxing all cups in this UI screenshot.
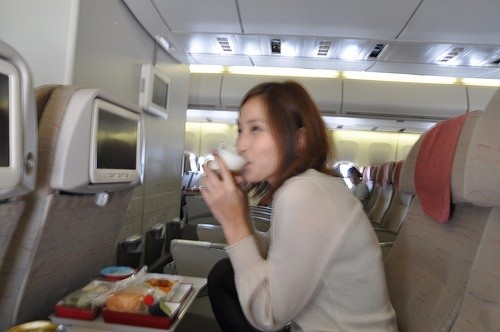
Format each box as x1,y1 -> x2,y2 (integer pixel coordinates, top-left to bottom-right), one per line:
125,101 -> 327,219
211,142 -> 247,181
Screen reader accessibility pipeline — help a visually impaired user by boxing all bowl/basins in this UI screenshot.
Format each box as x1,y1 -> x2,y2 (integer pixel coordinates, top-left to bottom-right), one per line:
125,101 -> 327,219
102,302 -> 181,328
54,280 -> 120,320
133,274 -> 183,300
100,266 -> 134,280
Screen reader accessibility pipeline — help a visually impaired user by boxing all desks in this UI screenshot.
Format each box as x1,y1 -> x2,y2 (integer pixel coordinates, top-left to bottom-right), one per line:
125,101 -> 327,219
48,273 -> 207,332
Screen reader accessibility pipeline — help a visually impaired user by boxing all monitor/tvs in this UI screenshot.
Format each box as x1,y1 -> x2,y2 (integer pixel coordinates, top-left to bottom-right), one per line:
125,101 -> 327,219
0,58 -> 23,189
90,99 -> 142,183
138,64 -> 171,119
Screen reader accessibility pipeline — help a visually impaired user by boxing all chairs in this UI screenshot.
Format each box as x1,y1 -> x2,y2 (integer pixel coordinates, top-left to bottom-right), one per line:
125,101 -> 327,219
169,88 -> 500,332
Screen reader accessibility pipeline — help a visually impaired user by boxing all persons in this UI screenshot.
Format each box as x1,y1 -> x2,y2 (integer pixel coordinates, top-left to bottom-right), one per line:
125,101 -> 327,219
348,166 -> 369,207
200,82 -> 398,332
